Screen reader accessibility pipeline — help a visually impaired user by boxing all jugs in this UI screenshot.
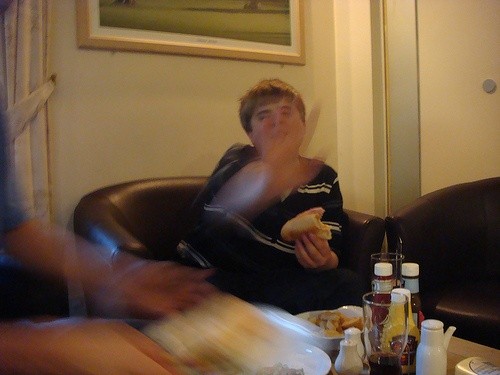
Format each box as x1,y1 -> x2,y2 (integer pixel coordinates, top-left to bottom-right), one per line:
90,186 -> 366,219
416,319 -> 456,374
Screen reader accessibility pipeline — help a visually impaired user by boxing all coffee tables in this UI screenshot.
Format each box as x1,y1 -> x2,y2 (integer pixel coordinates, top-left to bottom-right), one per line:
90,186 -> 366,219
328,334 -> 500,375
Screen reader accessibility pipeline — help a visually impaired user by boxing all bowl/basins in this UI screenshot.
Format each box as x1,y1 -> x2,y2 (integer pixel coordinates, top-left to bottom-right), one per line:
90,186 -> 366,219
294,305 -> 374,352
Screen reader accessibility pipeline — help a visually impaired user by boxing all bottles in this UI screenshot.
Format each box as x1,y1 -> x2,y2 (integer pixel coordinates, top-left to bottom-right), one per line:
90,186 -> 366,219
401,263 -> 422,333
372,263 -> 393,352
334,326 -> 367,375
382,287 -> 421,375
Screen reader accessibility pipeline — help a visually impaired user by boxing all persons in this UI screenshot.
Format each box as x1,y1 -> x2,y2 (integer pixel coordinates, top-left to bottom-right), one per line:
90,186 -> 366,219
181,78 -> 359,315
0,0 -> 217,375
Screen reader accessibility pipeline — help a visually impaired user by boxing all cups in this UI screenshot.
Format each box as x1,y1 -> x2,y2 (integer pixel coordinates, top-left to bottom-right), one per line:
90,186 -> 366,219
370,252 -> 404,291
362,291 -> 409,375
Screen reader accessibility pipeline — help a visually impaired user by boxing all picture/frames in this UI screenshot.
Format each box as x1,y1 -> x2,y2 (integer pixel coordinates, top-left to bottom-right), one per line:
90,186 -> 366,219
75,0 -> 306,66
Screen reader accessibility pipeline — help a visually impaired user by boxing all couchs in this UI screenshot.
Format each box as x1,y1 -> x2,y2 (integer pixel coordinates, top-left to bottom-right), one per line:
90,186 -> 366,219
72,176 -> 500,350
